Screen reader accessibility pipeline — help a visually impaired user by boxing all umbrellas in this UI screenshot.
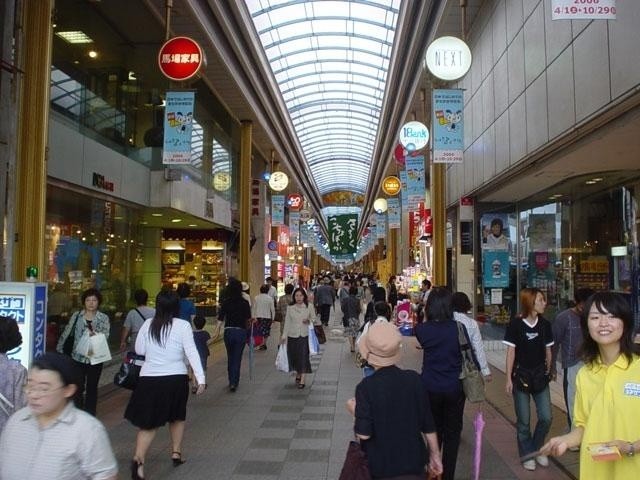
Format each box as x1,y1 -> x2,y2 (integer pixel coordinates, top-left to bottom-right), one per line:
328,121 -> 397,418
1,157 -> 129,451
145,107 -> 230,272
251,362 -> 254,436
472,402 -> 486,480
249,321 -> 255,387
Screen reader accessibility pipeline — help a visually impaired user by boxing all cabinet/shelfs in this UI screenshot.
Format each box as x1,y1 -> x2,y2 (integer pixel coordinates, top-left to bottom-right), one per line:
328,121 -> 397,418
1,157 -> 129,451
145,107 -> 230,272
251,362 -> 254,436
162,247 -> 226,288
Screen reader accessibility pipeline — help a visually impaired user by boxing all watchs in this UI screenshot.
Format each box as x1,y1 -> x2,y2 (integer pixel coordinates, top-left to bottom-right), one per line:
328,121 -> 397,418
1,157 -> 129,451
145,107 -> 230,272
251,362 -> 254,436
627,442 -> 635,457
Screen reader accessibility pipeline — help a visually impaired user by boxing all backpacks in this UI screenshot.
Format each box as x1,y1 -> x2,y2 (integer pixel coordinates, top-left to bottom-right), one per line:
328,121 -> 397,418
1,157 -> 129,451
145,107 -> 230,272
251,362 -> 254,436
342,313 -> 349,327
355,321 -> 372,368
274,300 -> 283,322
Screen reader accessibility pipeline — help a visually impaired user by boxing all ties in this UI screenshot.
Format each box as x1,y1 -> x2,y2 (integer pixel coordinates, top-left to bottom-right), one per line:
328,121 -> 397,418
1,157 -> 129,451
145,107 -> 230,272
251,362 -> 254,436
421,293 -> 425,303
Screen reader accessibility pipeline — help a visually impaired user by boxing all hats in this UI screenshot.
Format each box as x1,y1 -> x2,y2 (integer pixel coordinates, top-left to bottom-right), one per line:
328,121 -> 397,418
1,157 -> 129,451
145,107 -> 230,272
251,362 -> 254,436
323,277 -> 330,283
357,322 -> 403,366
241,282 -> 249,291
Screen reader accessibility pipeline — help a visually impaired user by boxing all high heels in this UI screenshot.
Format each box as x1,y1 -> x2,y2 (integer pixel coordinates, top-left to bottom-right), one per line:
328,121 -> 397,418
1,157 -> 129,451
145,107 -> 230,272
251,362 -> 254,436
230,383 -> 236,391
132,457 -> 144,480
172,452 -> 185,467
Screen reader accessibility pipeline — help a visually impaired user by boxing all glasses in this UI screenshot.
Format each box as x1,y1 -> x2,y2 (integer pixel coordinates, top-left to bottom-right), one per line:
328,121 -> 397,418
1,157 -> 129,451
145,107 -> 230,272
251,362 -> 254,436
22,384 -> 70,398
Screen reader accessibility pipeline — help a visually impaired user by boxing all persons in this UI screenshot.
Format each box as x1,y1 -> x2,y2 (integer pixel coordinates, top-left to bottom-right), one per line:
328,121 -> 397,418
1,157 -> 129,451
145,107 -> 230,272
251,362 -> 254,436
386,276 -> 397,311
291,275 -> 307,301
309,270 -> 379,287
215,280 -> 251,392
266,277 -> 277,307
277,284 -> 294,349
383,245 -> 387,259
358,302 -> 392,378
120,288 -> 156,362
177,282 -> 196,381
341,286 -> 361,352
241,281 -> 251,307
415,291 -> 486,480
365,287 -> 386,324
185,272 -> 196,284
313,278 -> 324,316
124,289 -> 205,480
191,315 -> 218,394
531,222 -> 552,248
410,280 -> 432,323
318,277 -> 335,326
282,288 -> 316,389
348,323 -> 443,480
487,219 -> 512,244
289,277 -> 294,286
482,225 -> 489,243
509,259 -> 528,316
560,282 -> 571,306
251,285 -> 275,350
453,292 -> 493,382
502,287 -> 554,470
360,288 -> 371,324
0,354 -> 119,480
56,288 -> 110,417
540,292 -> 640,480
0,316 -> 27,428
550,288 -> 595,452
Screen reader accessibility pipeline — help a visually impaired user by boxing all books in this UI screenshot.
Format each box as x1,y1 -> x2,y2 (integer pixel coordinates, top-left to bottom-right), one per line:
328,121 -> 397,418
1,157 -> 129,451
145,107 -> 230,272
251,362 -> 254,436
589,442 -> 622,462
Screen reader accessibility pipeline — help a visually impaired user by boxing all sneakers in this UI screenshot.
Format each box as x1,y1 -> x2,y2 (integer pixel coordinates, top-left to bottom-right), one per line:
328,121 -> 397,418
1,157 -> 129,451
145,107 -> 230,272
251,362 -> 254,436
523,459 -> 535,470
537,455 -> 548,467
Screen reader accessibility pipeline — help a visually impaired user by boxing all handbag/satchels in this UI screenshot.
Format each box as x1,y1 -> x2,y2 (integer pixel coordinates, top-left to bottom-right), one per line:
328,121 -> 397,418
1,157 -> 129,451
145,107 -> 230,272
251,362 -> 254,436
114,352 -> 146,390
339,428 -> 370,480
86,332 -> 112,366
275,343 -> 290,372
308,326 -> 320,354
315,325 -> 325,343
76,330 -> 91,357
64,310 -> 81,355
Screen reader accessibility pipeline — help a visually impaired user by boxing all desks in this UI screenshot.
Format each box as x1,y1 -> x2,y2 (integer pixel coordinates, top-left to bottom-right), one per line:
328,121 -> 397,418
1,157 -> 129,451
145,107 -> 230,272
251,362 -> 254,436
194,302 -> 219,316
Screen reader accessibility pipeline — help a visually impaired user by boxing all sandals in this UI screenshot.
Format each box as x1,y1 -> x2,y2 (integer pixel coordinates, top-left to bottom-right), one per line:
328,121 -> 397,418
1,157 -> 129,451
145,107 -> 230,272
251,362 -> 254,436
299,382 -> 304,388
296,376 -> 300,382
